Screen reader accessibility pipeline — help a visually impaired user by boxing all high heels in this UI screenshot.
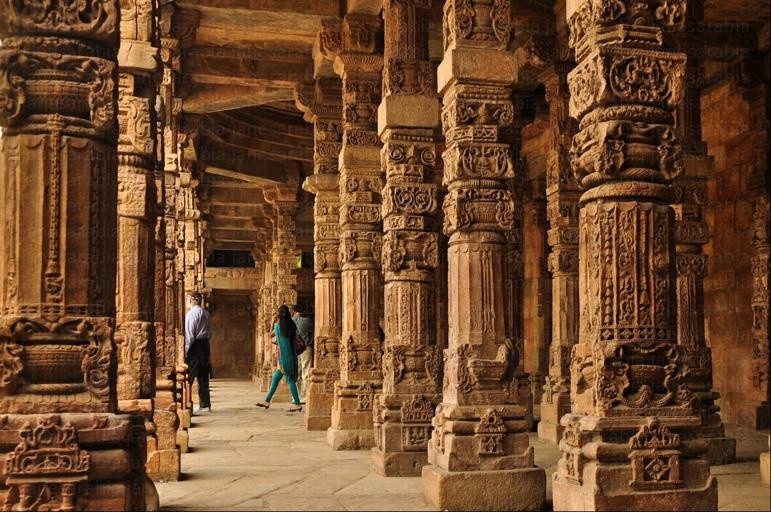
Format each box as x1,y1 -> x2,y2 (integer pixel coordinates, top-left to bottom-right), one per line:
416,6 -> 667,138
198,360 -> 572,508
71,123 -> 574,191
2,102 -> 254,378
256,402 -> 270,410
287,406 -> 303,412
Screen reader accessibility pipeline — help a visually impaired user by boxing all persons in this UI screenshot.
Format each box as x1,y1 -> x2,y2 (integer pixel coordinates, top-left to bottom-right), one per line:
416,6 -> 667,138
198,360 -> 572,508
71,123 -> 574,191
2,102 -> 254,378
256,305 -> 302,413
290,303 -> 315,404
185,290 -> 212,413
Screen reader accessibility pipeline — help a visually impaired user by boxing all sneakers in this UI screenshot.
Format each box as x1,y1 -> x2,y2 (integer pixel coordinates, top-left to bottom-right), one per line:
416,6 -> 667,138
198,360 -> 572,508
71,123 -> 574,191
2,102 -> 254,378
196,405 -> 211,413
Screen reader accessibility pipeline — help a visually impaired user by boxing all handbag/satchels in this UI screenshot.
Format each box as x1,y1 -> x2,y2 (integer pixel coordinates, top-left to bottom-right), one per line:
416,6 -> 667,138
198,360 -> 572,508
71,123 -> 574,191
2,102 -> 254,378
295,334 -> 308,356
